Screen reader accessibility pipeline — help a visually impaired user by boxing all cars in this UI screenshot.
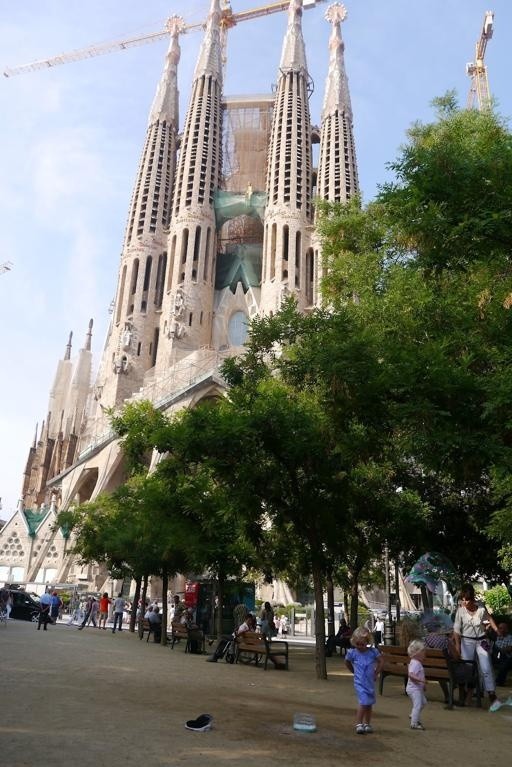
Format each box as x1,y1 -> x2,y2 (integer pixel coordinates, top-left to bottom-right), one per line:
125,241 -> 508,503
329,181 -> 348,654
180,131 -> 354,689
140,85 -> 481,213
371,609 -> 423,620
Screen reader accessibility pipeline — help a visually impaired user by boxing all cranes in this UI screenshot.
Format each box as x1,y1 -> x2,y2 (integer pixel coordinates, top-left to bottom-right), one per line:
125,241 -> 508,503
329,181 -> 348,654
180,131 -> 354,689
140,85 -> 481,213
458,8 -> 501,120
1,0 -> 330,94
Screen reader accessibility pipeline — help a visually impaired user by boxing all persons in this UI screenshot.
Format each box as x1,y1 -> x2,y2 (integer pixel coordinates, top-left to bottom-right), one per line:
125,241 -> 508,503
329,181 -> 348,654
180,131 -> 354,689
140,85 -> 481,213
325,616 -> 386,657
346,628 -> 384,734
206,601 -> 289,664
1,588 -> 125,634
404,640 -> 429,731
124,595 -> 215,647
400,584 -> 512,713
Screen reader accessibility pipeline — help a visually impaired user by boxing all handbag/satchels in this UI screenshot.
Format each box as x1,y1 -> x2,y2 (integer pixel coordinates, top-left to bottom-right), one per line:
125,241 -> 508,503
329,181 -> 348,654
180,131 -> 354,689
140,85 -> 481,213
228,641 -> 238,655
268,620 -> 275,630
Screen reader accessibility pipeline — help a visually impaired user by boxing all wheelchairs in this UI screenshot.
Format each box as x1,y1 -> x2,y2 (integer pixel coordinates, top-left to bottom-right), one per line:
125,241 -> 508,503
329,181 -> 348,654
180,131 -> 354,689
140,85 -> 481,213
225,632 -> 264,665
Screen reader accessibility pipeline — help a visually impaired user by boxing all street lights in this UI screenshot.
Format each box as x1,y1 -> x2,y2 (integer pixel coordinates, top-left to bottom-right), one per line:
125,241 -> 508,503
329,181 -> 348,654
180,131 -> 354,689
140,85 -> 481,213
380,538 -> 398,646
108,578 -> 113,599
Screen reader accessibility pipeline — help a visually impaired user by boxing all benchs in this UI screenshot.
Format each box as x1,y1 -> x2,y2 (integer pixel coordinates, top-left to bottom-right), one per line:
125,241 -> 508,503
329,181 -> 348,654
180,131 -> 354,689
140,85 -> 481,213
139,618 -> 156,642
171,621 -> 205,654
235,631 -> 289,671
375,645 -> 477,711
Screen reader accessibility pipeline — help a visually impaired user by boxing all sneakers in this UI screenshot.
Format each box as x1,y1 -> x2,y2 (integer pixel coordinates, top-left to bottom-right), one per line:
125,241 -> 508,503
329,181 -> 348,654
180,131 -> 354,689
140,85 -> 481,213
411,724 -> 424,730
356,724 -> 372,734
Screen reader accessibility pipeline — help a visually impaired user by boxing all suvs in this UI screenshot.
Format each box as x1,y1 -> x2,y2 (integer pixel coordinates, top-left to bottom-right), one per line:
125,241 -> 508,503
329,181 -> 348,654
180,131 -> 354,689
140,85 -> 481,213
1,587 -> 41,623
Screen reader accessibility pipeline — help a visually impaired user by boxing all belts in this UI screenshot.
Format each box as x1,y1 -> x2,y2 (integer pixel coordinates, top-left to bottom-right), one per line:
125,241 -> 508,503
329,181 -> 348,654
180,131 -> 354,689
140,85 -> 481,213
461,635 -> 486,640
